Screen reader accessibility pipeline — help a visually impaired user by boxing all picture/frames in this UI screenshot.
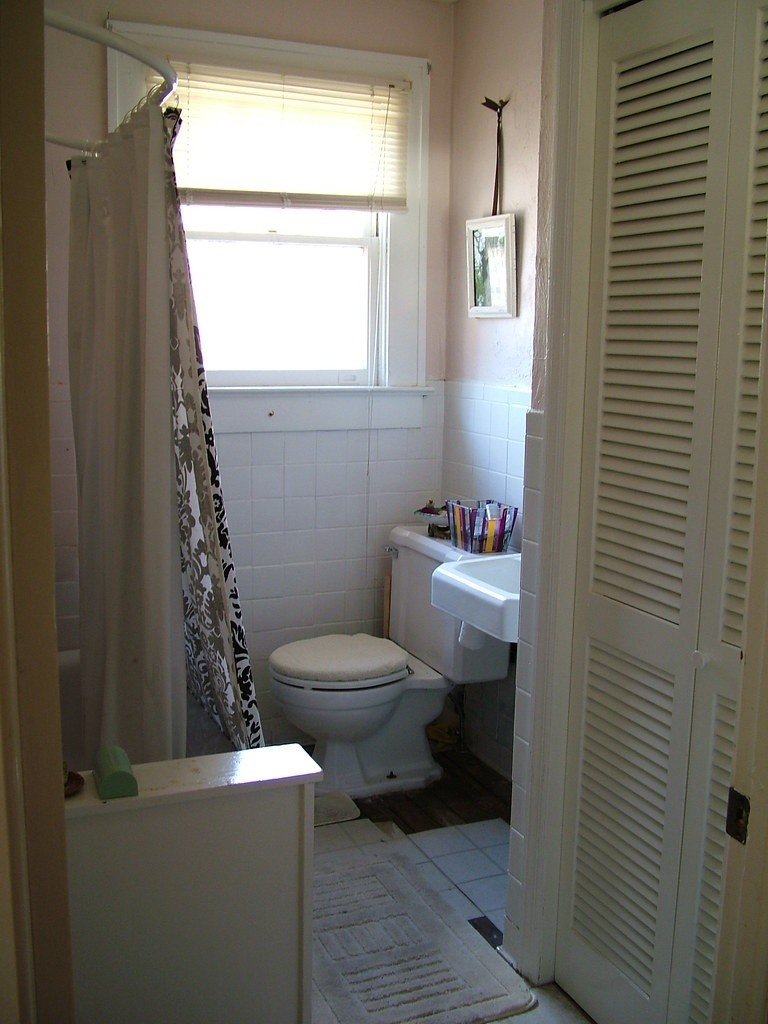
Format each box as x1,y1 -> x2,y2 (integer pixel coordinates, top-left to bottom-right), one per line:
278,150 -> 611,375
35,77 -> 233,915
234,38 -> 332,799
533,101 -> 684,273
465,213 -> 517,320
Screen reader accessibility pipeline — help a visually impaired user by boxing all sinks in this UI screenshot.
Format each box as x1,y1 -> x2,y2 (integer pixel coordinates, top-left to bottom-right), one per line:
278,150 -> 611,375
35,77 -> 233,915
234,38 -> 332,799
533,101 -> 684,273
429,552 -> 523,643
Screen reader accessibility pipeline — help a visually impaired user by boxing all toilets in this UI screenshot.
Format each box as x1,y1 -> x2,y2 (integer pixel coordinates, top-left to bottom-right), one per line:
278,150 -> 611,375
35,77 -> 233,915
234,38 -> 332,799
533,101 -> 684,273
267,521 -> 514,797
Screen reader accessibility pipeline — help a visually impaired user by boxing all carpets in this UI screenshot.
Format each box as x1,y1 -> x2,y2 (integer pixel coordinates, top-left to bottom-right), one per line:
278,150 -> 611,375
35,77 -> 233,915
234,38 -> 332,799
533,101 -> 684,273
310,850 -> 538,1024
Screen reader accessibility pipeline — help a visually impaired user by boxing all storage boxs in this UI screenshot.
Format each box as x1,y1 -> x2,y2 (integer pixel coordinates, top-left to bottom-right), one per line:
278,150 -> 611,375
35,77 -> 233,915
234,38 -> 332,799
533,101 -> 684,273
444,499 -> 519,554
